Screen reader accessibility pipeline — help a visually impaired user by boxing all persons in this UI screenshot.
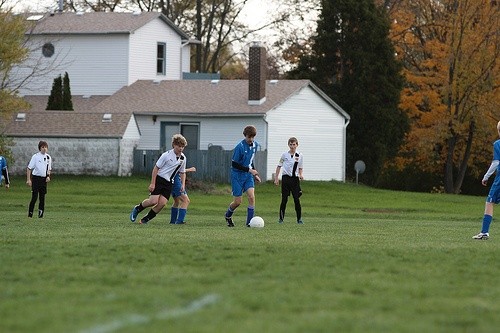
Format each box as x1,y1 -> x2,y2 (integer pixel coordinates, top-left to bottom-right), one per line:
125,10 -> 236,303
225,125 -> 262,227
0,148 -> 10,189
472,120 -> 500,240
130,134 -> 186,225
26,141 -> 52,220
169,167 -> 197,225
274,136 -> 304,225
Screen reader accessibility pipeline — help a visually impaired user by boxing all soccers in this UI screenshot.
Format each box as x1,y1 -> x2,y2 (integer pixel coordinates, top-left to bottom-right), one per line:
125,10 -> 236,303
250,216 -> 264,227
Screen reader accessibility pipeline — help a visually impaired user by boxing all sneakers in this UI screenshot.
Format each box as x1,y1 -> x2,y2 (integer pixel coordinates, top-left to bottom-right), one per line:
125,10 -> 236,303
298,219 -> 303,224
224,212 -> 234,227
139,216 -> 148,224
246,222 -> 250,227
279,219 -> 283,224
130,206 -> 138,222
169,220 -> 177,224
175,219 -> 186,224
472,232 -> 489,239
